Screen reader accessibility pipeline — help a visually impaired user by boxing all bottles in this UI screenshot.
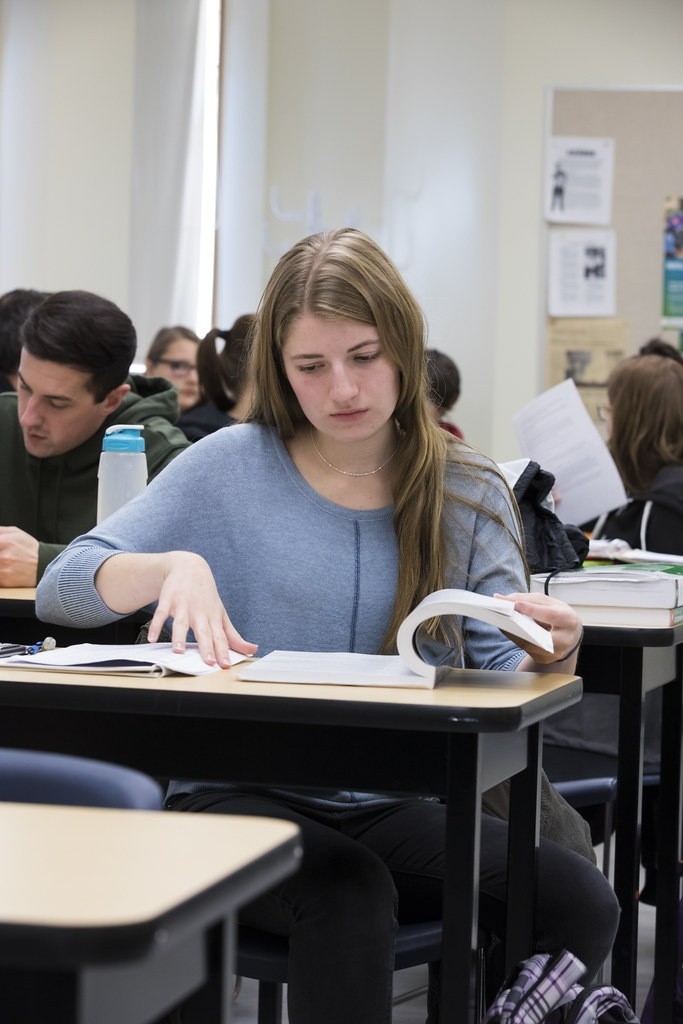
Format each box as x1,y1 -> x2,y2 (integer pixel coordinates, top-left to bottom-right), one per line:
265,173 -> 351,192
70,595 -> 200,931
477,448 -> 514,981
96,425 -> 148,526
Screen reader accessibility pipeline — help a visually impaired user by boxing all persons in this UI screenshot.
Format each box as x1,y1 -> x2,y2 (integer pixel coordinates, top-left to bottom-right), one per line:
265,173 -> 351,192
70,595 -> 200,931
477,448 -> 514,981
0,287 -> 190,753
542,337 -> 683,849
145,314 -> 261,445
34,227 -> 618,1023
421,347 -> 466,444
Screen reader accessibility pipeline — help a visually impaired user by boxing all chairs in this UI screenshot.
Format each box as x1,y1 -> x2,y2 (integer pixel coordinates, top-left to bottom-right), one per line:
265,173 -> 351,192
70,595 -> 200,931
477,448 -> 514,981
235,924 -> 442,1024
540,742 -> 660,986
0,751 -> 167,808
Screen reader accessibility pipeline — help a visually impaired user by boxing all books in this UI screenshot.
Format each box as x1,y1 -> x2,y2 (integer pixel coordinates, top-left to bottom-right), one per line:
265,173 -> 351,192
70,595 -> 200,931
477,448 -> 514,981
0,642 -> 185,677
237,589 -> 553,690
530,561 -> 683,627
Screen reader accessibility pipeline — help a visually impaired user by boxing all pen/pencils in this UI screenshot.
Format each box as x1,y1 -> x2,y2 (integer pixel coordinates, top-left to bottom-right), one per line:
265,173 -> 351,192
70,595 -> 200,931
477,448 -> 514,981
26,641 -> 43,655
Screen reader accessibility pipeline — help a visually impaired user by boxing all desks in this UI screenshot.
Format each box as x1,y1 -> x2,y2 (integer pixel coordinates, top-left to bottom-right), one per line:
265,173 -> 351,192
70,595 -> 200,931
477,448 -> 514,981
0,577 -> 683,1024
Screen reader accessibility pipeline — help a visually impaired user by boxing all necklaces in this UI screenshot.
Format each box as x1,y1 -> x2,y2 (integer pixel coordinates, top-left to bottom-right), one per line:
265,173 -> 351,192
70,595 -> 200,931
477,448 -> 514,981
310,427 -> 402,476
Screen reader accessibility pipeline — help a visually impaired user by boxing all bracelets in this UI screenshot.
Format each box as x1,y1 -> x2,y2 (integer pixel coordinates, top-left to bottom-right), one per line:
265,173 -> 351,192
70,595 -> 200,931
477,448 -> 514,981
556,630 -> 584,662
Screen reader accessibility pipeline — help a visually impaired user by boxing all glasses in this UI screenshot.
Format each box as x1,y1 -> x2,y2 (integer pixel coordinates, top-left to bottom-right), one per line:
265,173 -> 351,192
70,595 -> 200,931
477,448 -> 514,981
596,405 -> 612,423
157,357 -> 198,378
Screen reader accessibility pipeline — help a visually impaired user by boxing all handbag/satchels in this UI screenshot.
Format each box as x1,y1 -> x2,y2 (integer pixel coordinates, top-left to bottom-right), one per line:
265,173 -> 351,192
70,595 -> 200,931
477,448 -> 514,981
481,948 -> 640,1024
